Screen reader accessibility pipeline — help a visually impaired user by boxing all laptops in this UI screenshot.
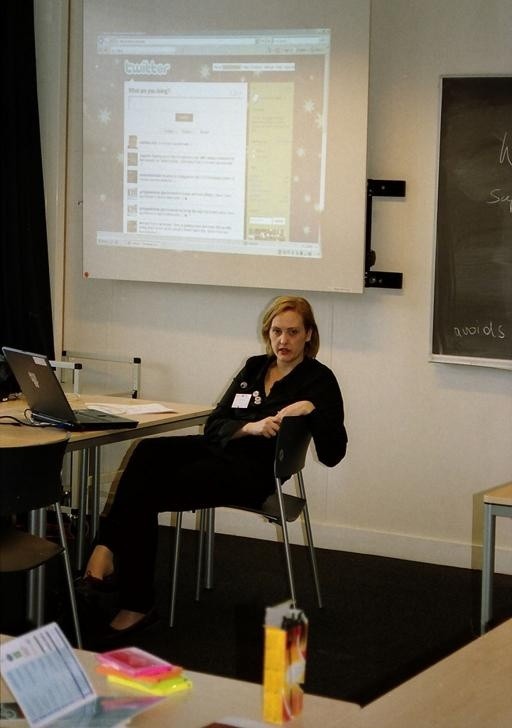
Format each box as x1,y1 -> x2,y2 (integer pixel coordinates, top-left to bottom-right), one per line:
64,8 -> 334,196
0,345 -> 138,431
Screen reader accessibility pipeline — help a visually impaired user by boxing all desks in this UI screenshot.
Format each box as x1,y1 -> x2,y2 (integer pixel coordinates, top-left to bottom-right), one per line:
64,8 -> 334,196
355,618 -> 512,728
0,388 -> 215,580
479,481 -> 512,635
0,633 -> 364,728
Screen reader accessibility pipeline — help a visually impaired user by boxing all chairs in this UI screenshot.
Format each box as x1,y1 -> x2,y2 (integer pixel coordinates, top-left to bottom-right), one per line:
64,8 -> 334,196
1,438 -> 88,646
165,409 -> 322,620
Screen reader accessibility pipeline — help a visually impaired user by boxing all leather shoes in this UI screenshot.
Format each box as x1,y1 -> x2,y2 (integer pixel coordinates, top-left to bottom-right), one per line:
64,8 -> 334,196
92,611 -> 164,650
75,561 -> 118,602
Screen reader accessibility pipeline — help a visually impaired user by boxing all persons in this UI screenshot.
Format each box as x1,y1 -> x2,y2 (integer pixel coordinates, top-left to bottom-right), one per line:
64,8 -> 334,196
77,294 -> 349,638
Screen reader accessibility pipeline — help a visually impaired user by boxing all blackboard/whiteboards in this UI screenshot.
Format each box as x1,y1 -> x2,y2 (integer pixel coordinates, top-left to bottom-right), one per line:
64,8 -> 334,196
425,74 -> 512,371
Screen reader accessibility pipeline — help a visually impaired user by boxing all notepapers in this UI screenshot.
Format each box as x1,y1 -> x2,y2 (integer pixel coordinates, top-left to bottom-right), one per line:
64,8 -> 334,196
97,647 -> 192,697
